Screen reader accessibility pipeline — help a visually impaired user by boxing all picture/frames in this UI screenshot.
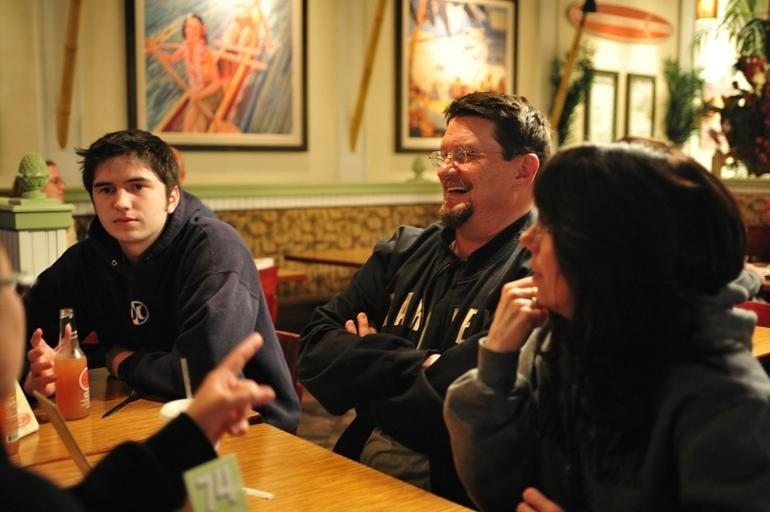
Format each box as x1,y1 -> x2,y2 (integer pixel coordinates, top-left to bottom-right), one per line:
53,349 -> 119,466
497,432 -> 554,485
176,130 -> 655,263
622,74 -> 657,139
584,71 -> 619,143
393,0 -> 519,154
123,0 -> 310,152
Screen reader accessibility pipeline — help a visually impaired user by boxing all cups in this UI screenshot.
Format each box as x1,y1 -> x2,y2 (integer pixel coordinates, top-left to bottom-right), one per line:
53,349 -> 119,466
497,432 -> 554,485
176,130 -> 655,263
159,399 -> 221,456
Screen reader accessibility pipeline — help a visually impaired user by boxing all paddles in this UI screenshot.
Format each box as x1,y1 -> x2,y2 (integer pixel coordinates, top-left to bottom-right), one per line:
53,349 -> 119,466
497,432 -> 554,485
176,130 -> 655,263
147,40 -> 242,133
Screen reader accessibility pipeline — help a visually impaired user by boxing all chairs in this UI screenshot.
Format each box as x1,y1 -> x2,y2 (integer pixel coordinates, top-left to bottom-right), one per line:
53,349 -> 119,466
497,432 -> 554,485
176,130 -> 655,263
275,328 -> 308,436
734,301 -> 770,328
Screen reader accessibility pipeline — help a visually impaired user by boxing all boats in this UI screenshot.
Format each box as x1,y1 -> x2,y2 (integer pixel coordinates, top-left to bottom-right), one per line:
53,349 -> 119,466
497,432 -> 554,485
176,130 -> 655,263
151,0 -> 266,131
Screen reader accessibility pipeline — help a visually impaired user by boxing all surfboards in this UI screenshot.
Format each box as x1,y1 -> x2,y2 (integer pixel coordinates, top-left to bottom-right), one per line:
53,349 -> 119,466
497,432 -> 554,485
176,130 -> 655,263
567,0 -> 672,43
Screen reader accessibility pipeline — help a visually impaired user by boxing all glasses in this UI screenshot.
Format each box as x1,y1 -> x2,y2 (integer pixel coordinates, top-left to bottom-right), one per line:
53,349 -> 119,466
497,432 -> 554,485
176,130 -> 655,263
427,144 -> 503,169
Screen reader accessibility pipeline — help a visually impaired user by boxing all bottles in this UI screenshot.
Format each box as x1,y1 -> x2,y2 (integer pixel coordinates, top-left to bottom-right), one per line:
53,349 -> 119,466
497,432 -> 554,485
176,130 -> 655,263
51,306 -> 91,421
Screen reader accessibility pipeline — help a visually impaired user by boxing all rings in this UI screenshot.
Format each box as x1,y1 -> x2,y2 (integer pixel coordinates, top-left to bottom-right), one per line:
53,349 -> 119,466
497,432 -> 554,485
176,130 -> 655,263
532,297 -> 538,304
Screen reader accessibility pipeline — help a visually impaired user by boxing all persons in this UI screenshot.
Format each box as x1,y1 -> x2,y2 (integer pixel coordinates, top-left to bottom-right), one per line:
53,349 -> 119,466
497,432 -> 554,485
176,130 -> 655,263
20,128 -> 300,436
296,91 -> 552,511
441,137 -> 769,511
620,136 -> 765,342
146,15 -> 223,130
0,242 -> 276,510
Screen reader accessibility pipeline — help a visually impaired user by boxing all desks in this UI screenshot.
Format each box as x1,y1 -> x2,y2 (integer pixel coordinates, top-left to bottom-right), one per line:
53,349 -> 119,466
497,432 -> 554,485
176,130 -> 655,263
285,246 -> 371,269
26,423 -> 476,511
8,366 -> 261,472
